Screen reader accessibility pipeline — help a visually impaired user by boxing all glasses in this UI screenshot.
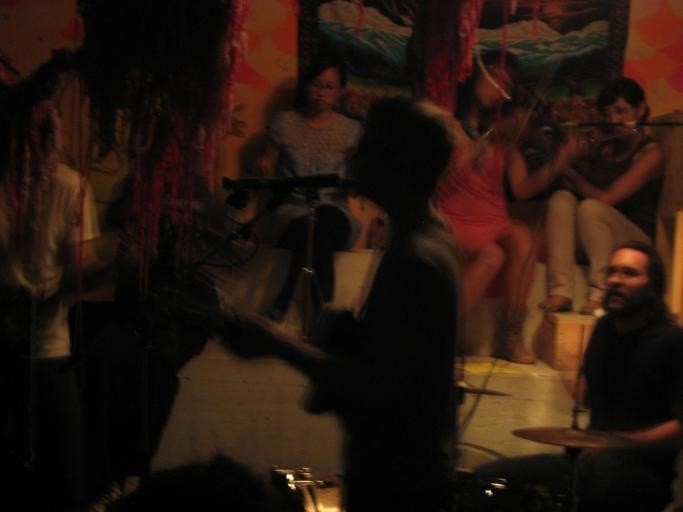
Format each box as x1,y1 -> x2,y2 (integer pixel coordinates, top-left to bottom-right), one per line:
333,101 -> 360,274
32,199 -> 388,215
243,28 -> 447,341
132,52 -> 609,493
599,266 -> 638,278
309,82 -> 341,93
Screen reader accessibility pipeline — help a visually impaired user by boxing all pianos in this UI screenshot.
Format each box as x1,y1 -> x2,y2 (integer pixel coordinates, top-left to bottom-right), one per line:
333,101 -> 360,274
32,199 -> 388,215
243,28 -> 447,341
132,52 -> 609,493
223,173 -> 353,188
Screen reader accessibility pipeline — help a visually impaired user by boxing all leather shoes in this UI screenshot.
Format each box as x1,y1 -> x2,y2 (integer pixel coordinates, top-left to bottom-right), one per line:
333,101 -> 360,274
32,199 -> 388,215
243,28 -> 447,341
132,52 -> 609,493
581,300 -> 601,313
537,296 -> 571,312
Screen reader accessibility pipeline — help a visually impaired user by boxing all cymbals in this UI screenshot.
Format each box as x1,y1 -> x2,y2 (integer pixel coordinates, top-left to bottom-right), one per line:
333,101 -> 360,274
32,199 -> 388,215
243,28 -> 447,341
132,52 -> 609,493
510,426 -> 630,449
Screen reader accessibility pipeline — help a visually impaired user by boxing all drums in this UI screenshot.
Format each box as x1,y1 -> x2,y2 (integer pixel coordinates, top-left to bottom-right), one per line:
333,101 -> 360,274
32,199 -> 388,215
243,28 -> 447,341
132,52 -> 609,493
268,466 -> 344,512
450,440 -> 582,511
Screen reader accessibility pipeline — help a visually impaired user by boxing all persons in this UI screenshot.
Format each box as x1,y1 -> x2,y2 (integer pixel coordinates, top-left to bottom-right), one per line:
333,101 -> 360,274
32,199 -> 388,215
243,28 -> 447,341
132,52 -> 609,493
0,45 -> 683,511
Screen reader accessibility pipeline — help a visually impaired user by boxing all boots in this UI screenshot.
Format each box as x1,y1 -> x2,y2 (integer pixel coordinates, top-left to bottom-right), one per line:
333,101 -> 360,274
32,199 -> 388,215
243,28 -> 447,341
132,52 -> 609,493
499,308 -> 538,364
